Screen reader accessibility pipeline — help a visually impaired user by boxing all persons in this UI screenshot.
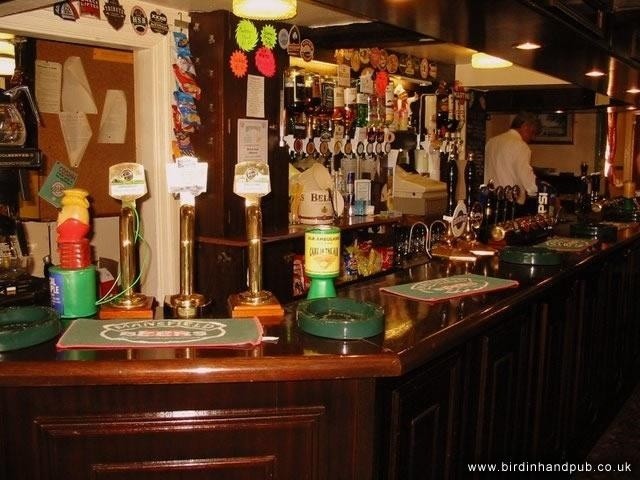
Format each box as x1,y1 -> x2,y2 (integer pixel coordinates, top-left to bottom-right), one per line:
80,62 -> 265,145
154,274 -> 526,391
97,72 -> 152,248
393,83 -> 419,128
484,111 -> 541,219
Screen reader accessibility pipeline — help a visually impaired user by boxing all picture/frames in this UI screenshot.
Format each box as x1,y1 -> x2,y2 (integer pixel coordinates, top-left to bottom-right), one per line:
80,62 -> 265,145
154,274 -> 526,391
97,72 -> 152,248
530,111 -> 574,145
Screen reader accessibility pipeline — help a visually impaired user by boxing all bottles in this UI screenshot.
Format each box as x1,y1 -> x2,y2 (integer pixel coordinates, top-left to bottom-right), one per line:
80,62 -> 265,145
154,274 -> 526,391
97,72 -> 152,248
395,225 -> 442,263
433,81 -> 465,145
280,67 -> 408,140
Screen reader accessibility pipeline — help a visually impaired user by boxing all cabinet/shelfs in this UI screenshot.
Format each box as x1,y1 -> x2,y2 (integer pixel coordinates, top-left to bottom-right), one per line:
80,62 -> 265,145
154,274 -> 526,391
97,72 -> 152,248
196,241 -> 294,314
187,9 -> 289,241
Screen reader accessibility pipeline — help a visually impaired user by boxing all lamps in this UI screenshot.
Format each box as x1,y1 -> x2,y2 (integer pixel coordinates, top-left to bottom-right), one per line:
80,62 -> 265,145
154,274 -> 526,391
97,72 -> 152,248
227,160 -> 285,318
160,156 -> 210,320
98,161 -> 156,322
471,52 -> 514,70
231,0 -> 298,21
